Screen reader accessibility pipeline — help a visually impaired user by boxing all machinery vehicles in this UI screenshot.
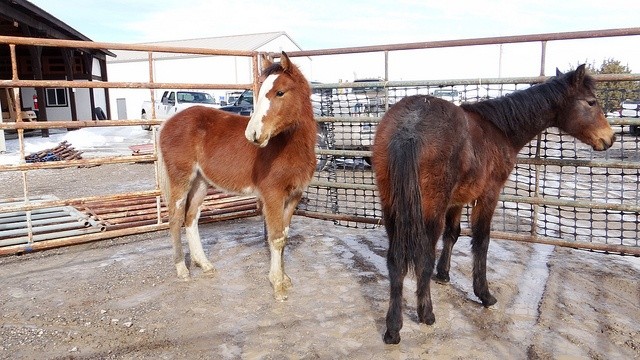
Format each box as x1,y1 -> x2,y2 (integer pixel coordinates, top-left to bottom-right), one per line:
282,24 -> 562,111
2,101 -> 37,133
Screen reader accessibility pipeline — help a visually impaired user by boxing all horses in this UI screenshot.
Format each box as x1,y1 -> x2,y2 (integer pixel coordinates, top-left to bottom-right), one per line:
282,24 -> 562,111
372,64 -> 616,343
157,52 -> 317,303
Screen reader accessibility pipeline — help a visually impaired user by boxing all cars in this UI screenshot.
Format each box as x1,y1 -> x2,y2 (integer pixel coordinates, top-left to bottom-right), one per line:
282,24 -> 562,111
429,89 -> 462,107
352,78 -> 387,96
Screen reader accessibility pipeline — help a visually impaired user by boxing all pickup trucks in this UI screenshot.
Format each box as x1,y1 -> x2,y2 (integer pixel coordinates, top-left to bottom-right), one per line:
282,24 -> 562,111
140,89 -> 220,131
220,79 -> 372,172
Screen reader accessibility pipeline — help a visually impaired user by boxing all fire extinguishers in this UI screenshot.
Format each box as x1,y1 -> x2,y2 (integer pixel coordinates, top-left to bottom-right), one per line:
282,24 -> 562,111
32,94 -> 39,109
